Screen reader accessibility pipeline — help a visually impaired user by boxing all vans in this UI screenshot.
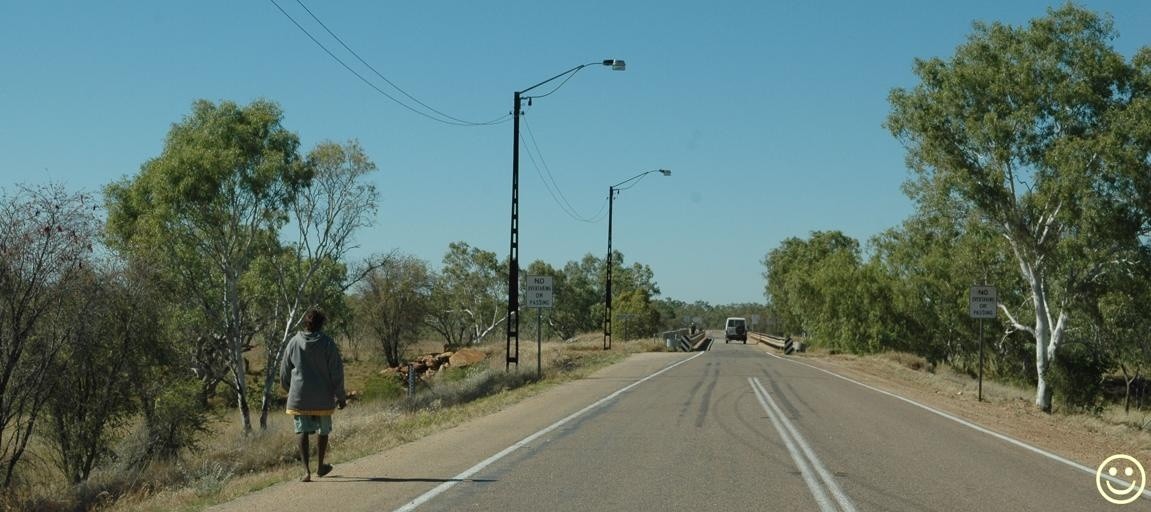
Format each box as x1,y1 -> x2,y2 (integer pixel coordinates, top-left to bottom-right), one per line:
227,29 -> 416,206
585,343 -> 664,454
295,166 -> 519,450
724,317 -> 747,345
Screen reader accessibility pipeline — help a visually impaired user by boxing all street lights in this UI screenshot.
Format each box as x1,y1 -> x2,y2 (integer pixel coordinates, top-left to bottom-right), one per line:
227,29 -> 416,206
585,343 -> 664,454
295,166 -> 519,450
602,169 -> 671,351
504,57 -> 626,371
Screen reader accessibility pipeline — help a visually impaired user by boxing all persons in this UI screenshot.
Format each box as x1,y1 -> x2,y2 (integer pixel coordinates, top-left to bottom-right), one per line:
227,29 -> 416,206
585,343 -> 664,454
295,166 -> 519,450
281,310 -> 349,484
691,323 -> 697,335
